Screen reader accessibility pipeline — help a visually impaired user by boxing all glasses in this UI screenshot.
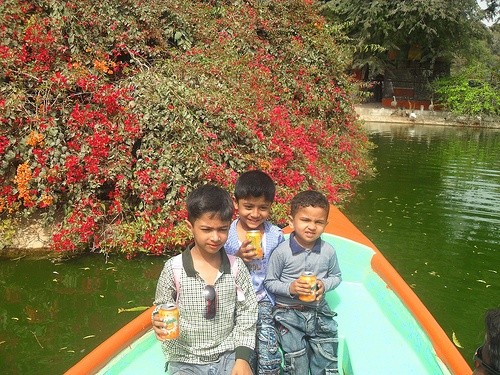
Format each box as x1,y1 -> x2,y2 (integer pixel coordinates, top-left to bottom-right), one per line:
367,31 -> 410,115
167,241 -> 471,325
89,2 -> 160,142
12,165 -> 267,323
472,345 -> 500,375
201,285 -> 217,320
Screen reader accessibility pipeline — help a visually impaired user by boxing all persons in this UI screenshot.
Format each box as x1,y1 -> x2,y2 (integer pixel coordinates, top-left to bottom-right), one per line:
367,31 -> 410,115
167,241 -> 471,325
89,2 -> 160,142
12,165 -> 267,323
263,190 -> 343,375
223,170 -> 285,375
152,185 -> 258,375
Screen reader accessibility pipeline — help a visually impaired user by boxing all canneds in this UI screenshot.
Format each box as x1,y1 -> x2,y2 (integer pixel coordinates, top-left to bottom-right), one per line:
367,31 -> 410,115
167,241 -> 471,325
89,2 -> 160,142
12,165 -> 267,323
245,230 -> 263,260
298,271 -> 316,302
159,304 -> 180,340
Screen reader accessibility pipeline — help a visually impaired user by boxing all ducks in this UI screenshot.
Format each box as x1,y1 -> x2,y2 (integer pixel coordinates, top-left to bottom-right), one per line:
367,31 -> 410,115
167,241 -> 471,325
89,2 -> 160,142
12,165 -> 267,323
429,98 -> 435,112
409,108 -> 417,125
390,95 -> 398,109
